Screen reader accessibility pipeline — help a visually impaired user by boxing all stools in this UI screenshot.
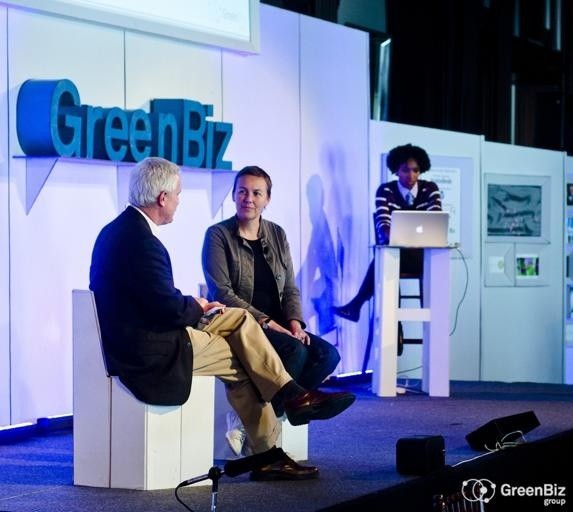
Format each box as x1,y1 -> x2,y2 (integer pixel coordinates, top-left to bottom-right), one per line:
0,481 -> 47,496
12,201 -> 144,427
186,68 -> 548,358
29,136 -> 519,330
361,270 -> 423,396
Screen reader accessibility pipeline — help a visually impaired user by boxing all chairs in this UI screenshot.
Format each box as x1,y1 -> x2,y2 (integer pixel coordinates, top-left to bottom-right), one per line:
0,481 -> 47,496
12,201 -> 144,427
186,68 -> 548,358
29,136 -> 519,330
200,285 -> 308,461
72,289 -> 215,490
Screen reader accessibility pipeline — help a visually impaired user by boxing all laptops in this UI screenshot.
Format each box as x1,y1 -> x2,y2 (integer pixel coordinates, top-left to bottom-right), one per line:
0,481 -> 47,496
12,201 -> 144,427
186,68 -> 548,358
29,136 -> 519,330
389,210 -> 449,249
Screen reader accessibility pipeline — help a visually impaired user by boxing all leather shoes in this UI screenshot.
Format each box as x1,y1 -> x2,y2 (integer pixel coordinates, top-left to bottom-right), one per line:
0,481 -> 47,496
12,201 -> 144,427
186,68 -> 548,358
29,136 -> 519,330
280,388 -> 356,427
252,453 -> 322,482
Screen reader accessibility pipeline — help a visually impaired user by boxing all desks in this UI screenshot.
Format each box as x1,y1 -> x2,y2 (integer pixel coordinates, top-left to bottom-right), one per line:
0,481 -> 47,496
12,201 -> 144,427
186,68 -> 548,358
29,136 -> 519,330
369,244 -> 458,397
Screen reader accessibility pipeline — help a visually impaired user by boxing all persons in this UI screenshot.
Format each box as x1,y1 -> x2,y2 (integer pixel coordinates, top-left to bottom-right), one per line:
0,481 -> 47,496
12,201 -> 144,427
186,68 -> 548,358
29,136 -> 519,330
331,142 -> 441,357
89,155 -> 356,482
200,164 -> 343,459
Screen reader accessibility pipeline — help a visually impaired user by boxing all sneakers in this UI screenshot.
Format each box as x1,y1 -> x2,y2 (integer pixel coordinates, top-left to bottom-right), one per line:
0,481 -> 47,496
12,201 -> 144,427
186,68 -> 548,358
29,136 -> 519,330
224,410 -> 246,457
327,302 -> 361,322
398,327 -> 406,356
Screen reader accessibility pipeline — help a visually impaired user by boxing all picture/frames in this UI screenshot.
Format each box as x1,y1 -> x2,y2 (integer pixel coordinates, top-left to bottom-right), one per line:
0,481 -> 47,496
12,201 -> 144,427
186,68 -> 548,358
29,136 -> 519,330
380,153 -> 474,259
484,173 -> 551,243
515,244 -> 551,287
484,244 -> 515,287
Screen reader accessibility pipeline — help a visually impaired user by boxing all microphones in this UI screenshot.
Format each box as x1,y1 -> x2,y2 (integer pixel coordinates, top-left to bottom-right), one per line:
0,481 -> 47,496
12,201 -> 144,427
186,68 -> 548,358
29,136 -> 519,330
224,446 -> 283,477
